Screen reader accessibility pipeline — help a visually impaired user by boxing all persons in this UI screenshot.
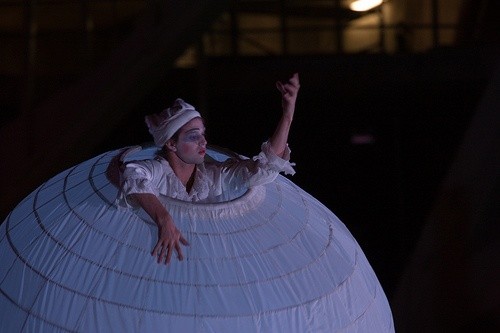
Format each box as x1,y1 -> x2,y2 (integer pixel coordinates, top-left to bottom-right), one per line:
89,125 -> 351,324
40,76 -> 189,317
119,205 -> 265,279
114,71 -> 301,265
